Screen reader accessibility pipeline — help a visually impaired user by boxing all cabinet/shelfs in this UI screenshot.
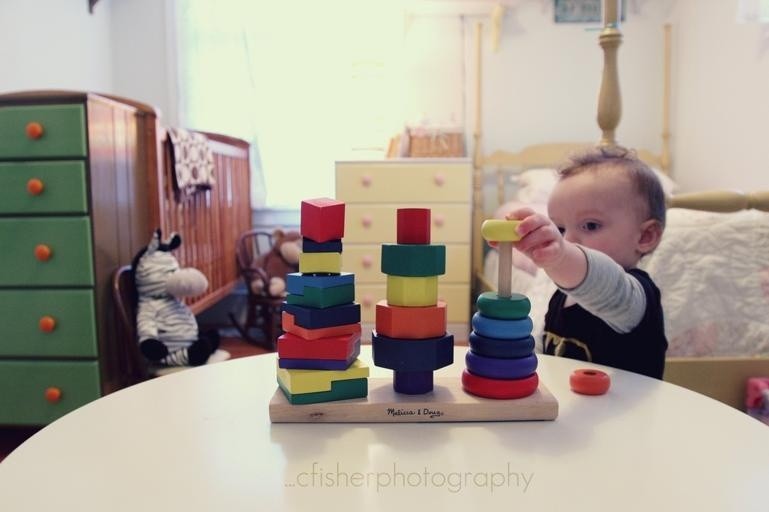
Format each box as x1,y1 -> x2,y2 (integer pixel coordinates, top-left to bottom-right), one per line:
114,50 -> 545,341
0,88 -> 147,427
334,159 -> 472,346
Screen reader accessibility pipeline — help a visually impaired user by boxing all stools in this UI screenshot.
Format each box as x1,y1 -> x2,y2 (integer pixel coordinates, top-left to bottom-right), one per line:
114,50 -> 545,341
110,263 -> 230,384
228,232 -> 289,352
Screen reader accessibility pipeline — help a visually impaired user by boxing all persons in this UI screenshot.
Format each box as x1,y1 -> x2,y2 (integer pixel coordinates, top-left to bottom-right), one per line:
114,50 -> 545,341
505,146 -> 668,381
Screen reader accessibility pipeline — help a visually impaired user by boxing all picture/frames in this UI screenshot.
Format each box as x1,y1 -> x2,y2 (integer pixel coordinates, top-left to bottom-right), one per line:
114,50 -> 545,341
547,1 -> 622,30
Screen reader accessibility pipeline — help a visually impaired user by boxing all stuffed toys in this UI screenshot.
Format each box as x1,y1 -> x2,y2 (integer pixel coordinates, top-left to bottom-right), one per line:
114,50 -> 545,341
277,197 -> 539,405
250,229 -> 302,297
569,369 -> 611,395
131,227 -> 221,367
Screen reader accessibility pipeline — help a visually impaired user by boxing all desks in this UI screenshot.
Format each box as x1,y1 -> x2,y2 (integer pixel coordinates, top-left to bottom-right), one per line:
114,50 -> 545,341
0,342 -> 768,512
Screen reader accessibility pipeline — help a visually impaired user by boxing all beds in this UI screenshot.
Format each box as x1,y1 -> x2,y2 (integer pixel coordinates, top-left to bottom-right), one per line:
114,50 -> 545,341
471,22 -> 767,411
92,93 -> 254,317
